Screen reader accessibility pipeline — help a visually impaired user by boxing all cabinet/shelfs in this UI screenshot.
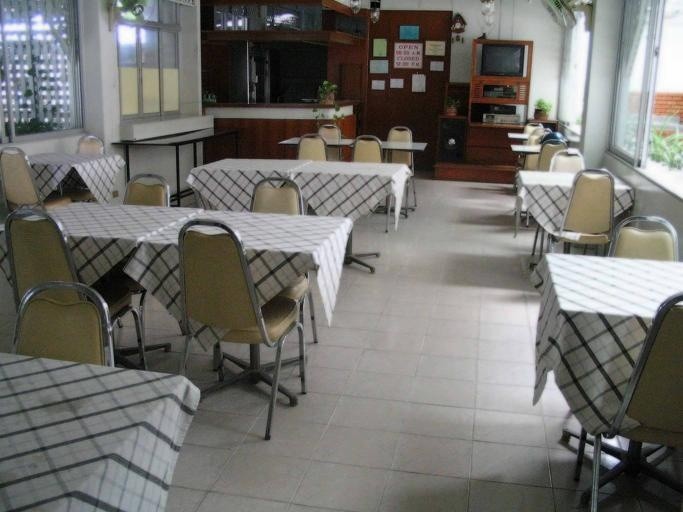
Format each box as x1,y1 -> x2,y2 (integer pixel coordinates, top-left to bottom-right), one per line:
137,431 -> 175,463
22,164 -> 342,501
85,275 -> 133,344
433,114 -> 558,185
467,37 -> 533,125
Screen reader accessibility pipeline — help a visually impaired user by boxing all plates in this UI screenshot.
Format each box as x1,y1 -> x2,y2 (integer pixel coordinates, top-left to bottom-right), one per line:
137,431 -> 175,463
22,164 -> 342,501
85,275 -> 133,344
301,99 -> 319,102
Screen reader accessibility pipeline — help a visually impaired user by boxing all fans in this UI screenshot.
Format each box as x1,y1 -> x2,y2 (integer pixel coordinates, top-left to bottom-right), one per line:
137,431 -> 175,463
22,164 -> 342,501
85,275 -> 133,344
106,1 -> 155,32
539,0 -> 593,32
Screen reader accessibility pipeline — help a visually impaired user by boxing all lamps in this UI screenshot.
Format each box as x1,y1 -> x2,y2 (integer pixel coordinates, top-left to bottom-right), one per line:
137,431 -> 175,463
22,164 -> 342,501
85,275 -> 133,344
370,1 -> 380,24
350,1 -> 361,16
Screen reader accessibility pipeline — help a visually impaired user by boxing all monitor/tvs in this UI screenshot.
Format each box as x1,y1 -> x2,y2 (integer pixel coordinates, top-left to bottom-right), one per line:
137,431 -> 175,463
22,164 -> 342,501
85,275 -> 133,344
479,43 -> 524,77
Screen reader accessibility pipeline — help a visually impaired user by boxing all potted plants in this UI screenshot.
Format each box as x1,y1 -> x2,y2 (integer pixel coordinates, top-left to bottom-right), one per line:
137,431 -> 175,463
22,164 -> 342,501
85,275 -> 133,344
533,99 -> 553,121
308,80 -> 345,131
445,97 -> 459,118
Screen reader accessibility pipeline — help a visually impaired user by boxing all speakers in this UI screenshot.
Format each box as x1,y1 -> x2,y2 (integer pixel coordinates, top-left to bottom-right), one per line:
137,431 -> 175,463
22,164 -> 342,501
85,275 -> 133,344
439,118 -> 466,164
